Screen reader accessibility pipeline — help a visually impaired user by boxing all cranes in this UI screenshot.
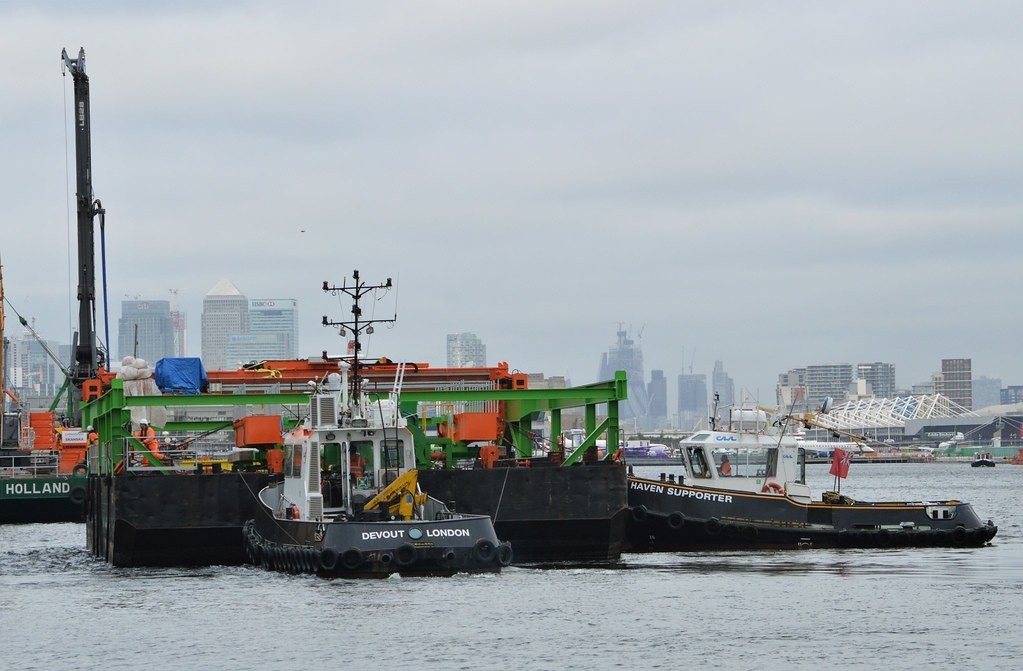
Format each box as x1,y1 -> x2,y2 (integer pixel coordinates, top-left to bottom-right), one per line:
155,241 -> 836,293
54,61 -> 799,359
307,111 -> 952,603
637,321 -> 644,344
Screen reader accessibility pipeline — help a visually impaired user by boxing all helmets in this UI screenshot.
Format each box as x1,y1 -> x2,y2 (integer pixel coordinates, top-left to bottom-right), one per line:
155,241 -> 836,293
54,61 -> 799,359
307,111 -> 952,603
87,425 -> 94,431
140,419 -> 148,424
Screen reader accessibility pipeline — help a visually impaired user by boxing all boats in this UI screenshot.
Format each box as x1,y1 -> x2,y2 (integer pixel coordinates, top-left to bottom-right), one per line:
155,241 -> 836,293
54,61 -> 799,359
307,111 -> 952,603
970,449 -> 996,468
241,269 -> 502,579
627,429 -> 999,550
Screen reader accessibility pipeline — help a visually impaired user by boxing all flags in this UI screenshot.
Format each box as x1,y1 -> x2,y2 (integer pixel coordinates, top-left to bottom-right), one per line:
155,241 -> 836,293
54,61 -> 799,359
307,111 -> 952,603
829,448 -> 854,479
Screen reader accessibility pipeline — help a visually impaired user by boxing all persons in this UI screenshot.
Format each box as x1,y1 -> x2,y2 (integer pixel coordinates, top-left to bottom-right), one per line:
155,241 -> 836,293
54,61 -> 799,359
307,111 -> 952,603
350,445 -> 366,478
719,455 -> 731,476
87,426 -> 98,444
135,418 -> 165,467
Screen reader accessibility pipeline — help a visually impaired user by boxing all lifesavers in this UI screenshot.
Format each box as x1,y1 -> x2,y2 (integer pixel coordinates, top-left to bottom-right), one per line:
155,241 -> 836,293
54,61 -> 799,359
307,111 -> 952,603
762,482 -> 783,501
292,506 -> 300,519
69,485 -> 88,506
377,550 -> 394,567
72,464 -> 88,479
394,541 -> 419,567
742,524 -> 759,541
496,544 -> 514,567
834,525 -> 989,549
666,510 -> 686,531
724,522 -> 741,539
241,520 -> 364,577
472,537 -> 497,563
760,429 -> 765,435
704,516 -> 722,537
632,504 -> 648,523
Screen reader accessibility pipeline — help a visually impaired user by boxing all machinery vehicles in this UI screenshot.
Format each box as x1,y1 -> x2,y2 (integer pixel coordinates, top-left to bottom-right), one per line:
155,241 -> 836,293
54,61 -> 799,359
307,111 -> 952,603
0,47 -> 152,476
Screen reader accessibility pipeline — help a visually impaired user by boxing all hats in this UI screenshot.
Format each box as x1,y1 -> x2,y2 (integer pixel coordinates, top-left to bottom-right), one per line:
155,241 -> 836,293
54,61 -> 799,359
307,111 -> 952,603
349,446 -> 356,452
